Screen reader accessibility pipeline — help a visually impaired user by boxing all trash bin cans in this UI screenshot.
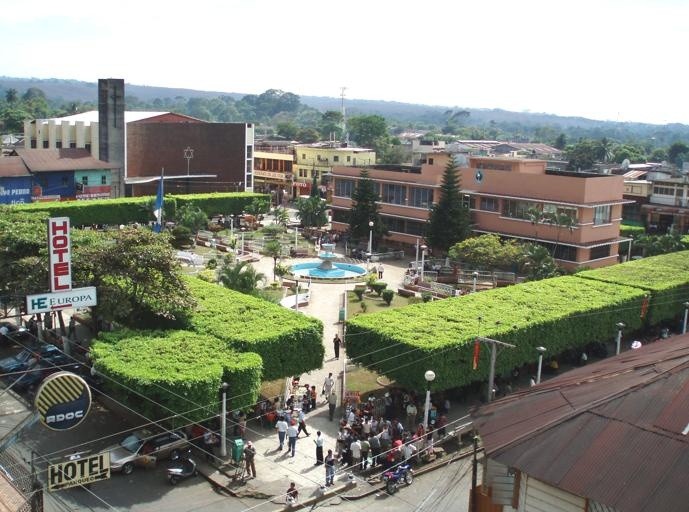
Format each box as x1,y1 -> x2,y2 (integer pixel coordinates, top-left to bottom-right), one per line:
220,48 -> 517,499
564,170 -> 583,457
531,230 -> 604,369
233,440 -> 244,462
339,310 -> 345,323
431,410 -> 436,420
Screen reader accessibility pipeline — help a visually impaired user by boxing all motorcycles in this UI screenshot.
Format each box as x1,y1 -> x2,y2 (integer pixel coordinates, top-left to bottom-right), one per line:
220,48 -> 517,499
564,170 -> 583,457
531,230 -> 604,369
160,455 -> 198,483
382,463 -> 416,494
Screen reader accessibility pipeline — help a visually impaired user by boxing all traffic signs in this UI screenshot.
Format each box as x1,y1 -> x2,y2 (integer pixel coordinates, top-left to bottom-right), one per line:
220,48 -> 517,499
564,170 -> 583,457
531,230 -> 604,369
46,450 -> 111,491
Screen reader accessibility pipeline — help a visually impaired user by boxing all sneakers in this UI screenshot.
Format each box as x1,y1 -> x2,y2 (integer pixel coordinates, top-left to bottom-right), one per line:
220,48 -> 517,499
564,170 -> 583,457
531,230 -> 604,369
248,474 -> 256,478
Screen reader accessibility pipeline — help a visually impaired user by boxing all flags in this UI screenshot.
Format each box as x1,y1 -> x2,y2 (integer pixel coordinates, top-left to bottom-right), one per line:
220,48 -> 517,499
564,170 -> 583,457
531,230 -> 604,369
152,179 -> 164,232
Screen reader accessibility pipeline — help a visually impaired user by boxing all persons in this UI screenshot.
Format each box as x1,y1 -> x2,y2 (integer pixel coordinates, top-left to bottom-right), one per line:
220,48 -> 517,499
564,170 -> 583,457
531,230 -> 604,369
29,315 -> 35,335
333,333 -> 341,360
505,368 -> 537,393
67,316 -> 76,338
0,324 -> 10,345
191,373 -> 450,504
70,450 -> 81,460
549,356 -> 558,371
424,262 -> 441,276
378,263 -> 384,279
579,351 -> 587,364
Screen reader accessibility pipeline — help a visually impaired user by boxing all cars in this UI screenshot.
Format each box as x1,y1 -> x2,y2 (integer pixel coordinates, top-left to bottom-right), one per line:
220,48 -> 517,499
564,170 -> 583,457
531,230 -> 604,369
102,428 -> 195,476
0,322 -> 79,392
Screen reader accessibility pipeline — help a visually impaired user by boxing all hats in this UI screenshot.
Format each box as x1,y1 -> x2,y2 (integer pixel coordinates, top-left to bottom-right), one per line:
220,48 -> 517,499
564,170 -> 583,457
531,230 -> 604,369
247,441 -> 252,446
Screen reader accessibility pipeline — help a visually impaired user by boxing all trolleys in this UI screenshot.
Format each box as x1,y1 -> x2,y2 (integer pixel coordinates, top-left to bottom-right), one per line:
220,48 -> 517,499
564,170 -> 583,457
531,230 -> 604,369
231,452 -> 251,487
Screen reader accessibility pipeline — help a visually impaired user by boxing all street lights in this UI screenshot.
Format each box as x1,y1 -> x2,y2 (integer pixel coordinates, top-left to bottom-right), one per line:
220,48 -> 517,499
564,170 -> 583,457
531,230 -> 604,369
421,369 -> 436,434
219,384 -> 230,459
419,244 -> 428,280
535,345 -> 546,384
291,274 -> 301,314
471,272 -> 482,292
682,302 -> 689,334
615,322 -> 625,355
365,221 -> 378,275
